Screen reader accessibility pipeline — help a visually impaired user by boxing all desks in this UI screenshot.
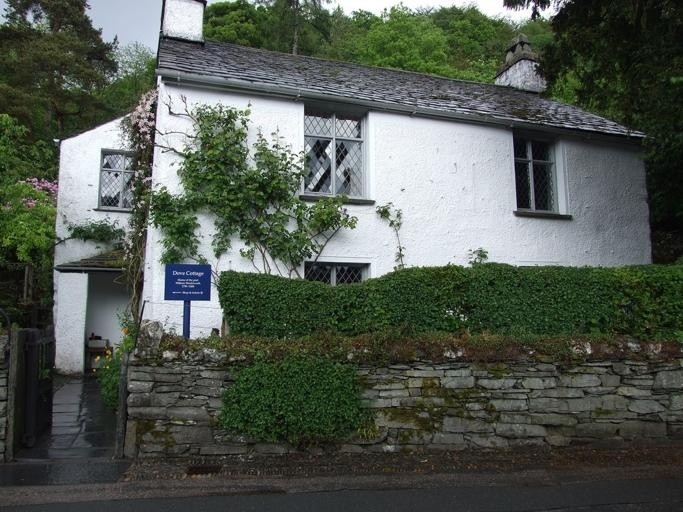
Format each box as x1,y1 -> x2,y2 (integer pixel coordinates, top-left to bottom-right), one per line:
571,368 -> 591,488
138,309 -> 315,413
86,346 -> 113,372
